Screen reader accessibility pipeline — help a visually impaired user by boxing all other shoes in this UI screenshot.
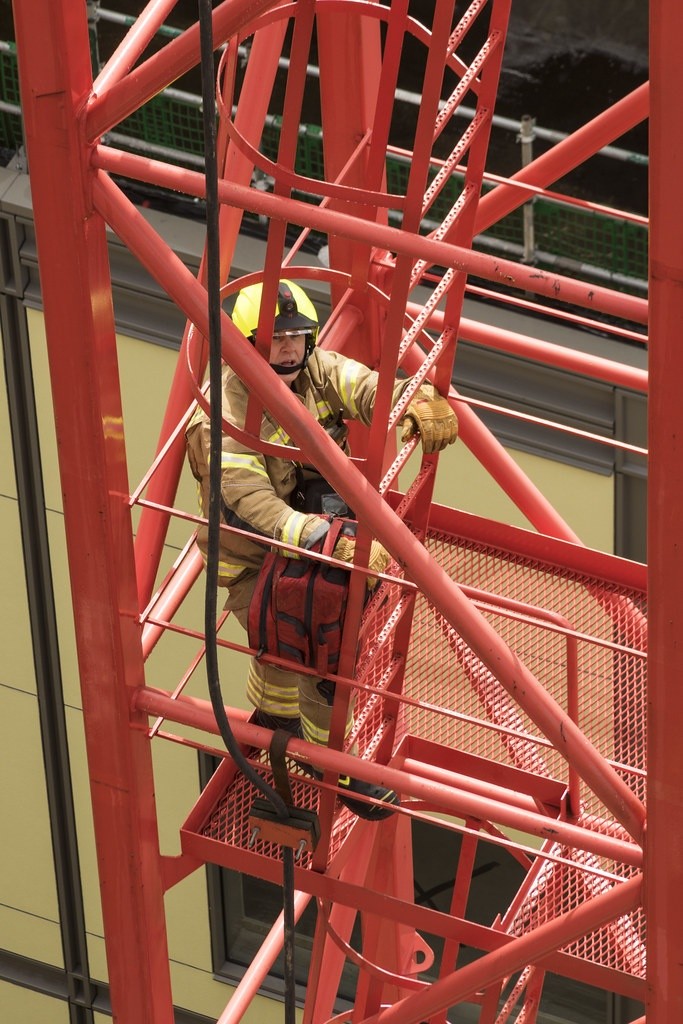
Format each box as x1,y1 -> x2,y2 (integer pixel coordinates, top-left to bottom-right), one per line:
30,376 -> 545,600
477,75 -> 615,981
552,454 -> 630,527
256,711 -> 323,781
314,770 -> 399,819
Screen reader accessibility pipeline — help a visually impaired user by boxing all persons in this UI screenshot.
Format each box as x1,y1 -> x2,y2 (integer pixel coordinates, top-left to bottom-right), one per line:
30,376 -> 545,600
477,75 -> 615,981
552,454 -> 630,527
186,281 -> 461,820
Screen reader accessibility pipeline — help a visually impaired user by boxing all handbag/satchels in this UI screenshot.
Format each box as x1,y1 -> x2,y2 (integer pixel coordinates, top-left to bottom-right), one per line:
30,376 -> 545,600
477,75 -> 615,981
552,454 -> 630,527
290,469 -> 350,517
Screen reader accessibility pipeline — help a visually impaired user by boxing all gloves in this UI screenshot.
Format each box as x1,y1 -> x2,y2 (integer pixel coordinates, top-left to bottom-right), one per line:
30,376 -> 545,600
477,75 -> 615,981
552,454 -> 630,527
332,537 -> 391,590
401,384 -> 458,453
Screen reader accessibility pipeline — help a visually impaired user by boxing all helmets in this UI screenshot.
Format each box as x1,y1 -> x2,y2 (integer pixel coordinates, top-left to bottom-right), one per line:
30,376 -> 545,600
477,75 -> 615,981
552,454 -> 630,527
231,278 -> 320,347
